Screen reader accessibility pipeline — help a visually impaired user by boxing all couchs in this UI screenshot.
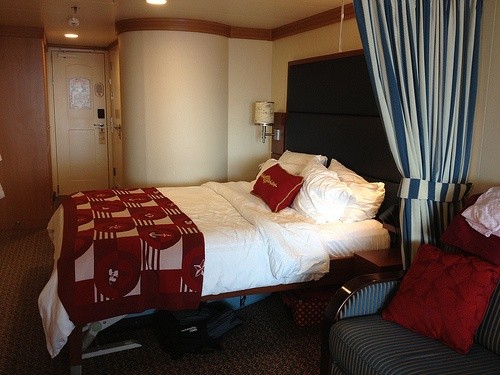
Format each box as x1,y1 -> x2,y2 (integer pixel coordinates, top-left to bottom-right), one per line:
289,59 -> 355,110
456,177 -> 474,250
320,195 -> 500,375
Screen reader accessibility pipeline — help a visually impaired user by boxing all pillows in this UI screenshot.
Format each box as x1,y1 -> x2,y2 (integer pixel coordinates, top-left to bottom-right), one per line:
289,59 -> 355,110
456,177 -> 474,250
382,243 -> 500,355
250,150 -> 385,225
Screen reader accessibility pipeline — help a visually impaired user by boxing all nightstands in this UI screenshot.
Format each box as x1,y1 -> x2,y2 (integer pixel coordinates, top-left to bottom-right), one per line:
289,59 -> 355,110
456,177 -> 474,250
354,249 -> 402,276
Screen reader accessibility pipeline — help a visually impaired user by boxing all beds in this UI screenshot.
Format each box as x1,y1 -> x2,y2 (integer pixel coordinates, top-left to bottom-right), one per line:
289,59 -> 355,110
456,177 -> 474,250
37,175 -> 394,375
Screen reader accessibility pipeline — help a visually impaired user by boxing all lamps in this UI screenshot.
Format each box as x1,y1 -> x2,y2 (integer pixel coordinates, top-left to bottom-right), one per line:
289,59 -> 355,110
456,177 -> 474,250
65,18 -> 79,38
253,101 -> 281,143
